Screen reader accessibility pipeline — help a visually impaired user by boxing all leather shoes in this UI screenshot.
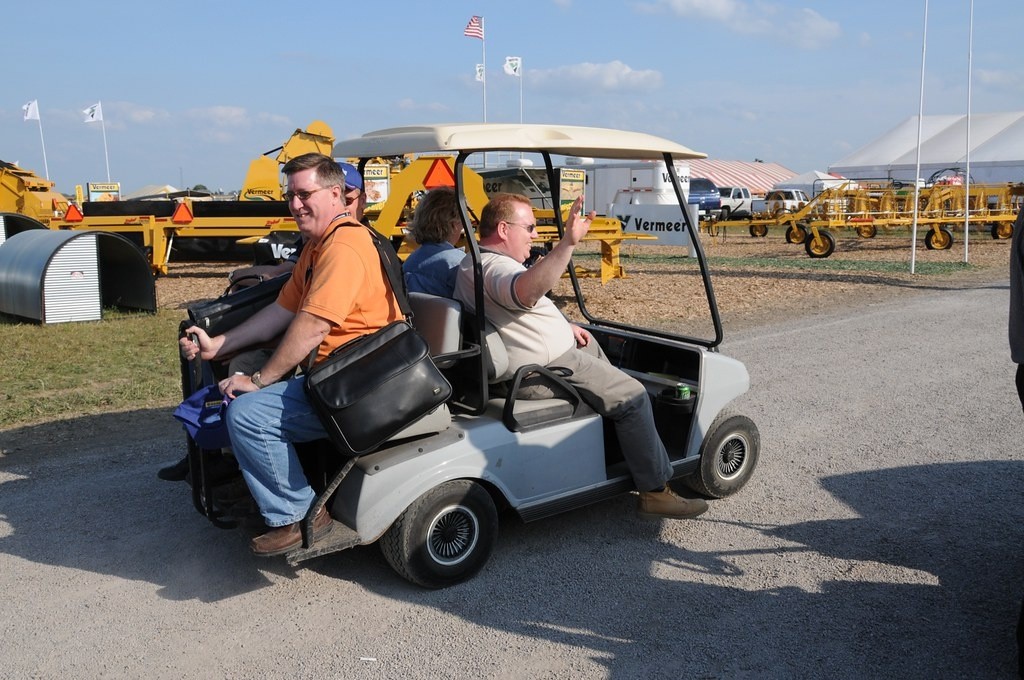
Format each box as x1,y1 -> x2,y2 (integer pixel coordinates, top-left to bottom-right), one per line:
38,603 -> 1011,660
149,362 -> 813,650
230,498 -> 260,519
249,503 -> 334,557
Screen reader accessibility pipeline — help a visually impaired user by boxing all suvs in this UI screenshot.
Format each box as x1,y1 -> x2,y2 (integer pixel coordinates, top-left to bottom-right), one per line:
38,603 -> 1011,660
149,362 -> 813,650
688,178 -> 722,221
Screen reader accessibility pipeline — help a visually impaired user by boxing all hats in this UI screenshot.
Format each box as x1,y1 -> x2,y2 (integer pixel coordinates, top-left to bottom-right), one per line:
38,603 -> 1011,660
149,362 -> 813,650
172,384 -> 232,449
337,161 -> 362,190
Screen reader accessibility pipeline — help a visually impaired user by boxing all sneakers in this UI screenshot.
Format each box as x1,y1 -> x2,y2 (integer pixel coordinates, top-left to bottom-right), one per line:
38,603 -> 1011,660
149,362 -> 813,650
636,482 -> 709,520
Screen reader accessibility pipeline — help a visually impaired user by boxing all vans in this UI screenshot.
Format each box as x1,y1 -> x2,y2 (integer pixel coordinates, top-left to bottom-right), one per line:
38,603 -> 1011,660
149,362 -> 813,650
716,186 -> 752,222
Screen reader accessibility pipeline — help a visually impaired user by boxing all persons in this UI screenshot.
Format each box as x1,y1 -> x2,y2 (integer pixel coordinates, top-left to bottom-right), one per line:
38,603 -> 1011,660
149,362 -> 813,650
458,192 -> 708,522
1009,206 -> 1024,416
158,152 -> 465,554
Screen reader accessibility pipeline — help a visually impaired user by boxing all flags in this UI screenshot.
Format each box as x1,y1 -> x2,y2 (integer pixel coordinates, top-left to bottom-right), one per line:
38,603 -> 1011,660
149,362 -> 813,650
83,100 -> 104,122
23,98 -> 41,121
504,56 -> 520,75
464,16 -> 484,38
476,63 -> 484,80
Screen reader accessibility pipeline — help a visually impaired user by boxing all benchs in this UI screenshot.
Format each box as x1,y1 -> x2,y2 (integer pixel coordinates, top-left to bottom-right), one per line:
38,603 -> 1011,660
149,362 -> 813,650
454,298 -> 595,432
386,293 -> 480,443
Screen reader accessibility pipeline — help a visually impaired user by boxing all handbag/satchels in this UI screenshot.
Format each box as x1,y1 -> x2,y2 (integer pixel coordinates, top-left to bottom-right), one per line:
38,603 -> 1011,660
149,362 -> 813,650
304,320 -> 453,453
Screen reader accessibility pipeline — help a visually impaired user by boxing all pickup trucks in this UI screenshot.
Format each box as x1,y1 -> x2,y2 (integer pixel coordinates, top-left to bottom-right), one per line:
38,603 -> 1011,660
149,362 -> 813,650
750,189 -> 813,217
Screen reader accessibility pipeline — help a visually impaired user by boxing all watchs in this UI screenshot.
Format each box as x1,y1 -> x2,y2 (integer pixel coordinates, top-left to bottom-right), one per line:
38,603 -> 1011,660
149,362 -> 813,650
251,371 -> 264,389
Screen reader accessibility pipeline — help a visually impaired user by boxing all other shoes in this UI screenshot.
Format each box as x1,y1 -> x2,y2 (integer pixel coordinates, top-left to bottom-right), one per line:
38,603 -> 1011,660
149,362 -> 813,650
214,478 -> 251,503
157,454 -> 190,481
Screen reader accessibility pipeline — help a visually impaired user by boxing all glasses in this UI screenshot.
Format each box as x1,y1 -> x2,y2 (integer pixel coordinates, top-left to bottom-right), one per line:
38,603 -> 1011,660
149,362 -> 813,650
495,222 -> 536,234
282,185 -> 343,200
345,195 -> 360,206
